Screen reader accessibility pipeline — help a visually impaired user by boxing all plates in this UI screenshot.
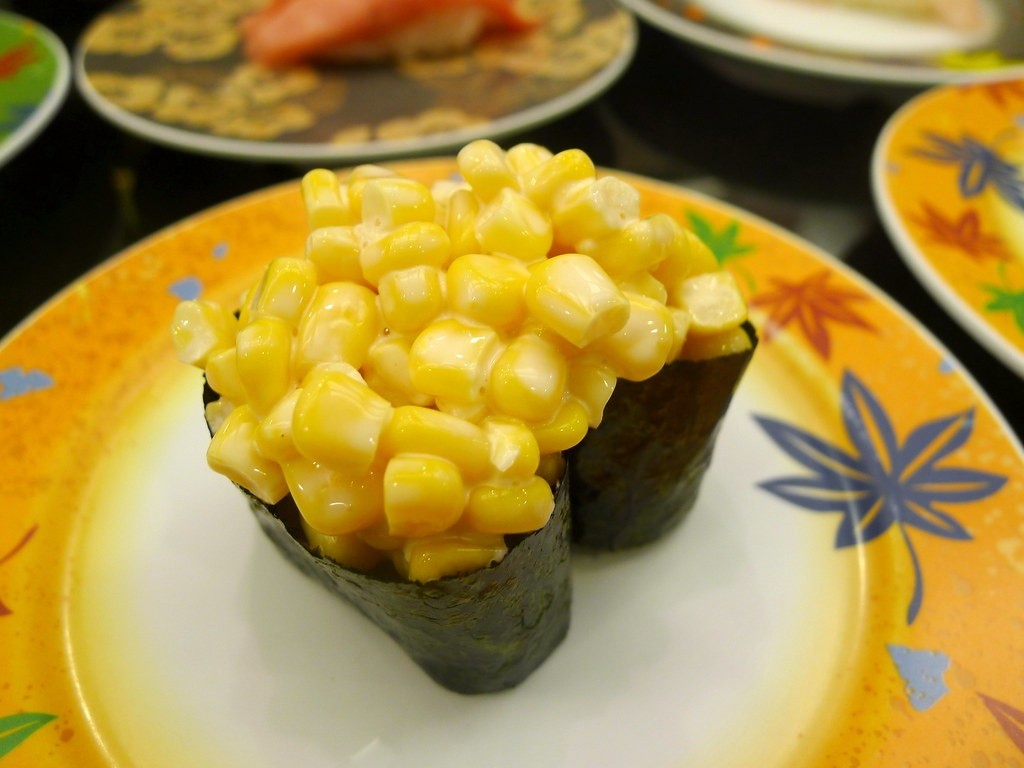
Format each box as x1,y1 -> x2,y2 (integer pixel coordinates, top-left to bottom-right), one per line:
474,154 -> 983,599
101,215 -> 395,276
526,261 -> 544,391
869,69 -> 1024,378
0,154 -> 1024,768
0,14 -> 72,166
73,1 -> 638,164
621,0 -> 1024,110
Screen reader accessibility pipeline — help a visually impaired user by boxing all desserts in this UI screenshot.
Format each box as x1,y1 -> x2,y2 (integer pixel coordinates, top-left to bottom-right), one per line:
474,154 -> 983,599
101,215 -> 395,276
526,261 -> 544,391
165,140 -> 759,697
